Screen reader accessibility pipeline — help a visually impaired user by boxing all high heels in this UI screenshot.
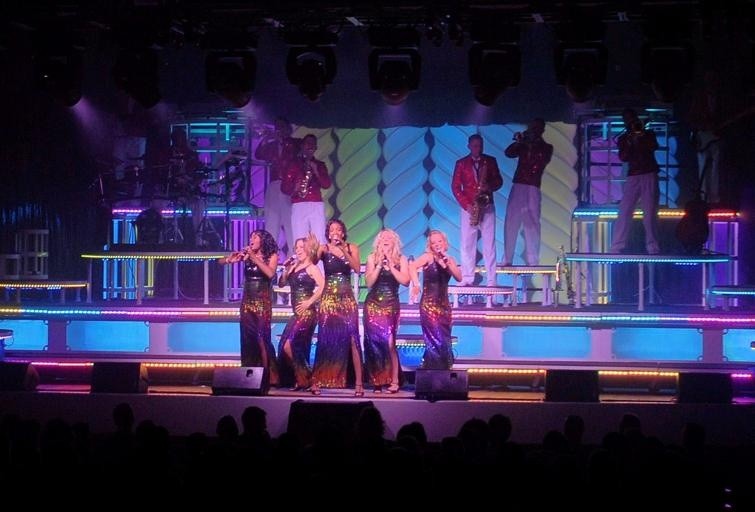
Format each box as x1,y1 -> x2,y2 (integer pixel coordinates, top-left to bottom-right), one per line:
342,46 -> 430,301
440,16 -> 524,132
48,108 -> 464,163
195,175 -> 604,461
311,390 -> 321,395
355,384 -> 364,397
373,385 -> 400,394
289,386 -> 305,392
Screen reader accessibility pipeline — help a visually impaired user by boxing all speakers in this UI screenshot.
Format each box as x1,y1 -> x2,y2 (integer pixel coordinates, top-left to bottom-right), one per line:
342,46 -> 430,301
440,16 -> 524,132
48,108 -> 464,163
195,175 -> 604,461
0,361 -> 40,390
212,367 -> 271,395
89,362 -> 148,393
676,371 -> 733,404
543,369 -> 600,402
414,369 -> 469,403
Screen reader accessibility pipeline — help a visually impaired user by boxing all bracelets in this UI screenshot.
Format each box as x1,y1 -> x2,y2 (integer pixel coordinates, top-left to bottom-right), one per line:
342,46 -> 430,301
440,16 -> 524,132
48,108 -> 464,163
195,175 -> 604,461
225,257 -> 229,264
376,265 -> 382,269
282,269 -> 288,273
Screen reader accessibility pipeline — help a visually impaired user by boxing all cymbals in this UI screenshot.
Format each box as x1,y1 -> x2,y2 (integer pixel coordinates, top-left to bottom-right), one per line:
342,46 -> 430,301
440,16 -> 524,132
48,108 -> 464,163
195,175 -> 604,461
193,167 -> 218,173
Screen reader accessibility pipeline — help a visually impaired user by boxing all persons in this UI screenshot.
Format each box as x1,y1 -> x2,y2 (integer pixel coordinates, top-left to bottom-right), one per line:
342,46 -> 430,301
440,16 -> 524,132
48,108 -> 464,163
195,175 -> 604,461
364,229 -> 410,394
452,135 -> 503,287
278,238 -> 325,391
501,118 -> 553,267
256,119 -> 301,260
684,80 -> 737,203
281,134 -> 331,276
305,219 -> 364,397
610,109 -> 660,255
219,231 -> 278,387
408,230 -> 462,370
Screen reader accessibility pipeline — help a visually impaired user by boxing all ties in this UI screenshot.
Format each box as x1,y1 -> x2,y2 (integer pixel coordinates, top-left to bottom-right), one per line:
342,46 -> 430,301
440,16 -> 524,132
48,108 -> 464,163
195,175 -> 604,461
473,162 -> 480,181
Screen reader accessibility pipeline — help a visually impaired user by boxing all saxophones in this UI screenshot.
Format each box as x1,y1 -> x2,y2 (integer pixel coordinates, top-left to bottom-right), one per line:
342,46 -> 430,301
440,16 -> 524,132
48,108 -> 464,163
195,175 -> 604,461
470,152 -> 490,226
299,150 -> 314,198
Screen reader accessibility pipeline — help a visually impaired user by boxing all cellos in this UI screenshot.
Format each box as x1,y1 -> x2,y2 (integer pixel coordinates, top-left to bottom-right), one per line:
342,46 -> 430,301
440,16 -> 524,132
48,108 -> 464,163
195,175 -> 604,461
678,160 -> 709,248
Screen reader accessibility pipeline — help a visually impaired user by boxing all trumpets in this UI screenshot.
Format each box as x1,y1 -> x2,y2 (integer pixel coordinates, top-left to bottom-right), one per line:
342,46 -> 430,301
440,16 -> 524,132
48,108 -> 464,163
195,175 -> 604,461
257,130 -> 286,138
512,130 -> 540,141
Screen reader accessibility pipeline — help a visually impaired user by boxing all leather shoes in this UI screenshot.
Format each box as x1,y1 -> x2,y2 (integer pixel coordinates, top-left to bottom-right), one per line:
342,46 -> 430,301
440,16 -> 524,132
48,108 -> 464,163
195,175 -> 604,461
487,281 -> 496,288
456,279 -> 474,287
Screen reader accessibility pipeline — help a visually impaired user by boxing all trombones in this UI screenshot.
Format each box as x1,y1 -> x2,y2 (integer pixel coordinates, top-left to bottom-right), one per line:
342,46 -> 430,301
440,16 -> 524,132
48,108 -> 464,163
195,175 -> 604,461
612,118 -> 651,145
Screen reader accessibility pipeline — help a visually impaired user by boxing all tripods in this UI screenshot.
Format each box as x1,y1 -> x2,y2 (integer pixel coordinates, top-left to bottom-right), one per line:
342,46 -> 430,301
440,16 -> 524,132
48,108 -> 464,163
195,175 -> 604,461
195,200 -> 223,249
163,202 -> 184,243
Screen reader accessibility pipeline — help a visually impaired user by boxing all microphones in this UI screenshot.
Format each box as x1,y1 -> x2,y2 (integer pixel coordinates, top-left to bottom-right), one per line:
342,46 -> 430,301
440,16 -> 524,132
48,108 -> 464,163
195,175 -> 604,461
436,247 -> 448,264
284,255 -> 296,268
334,234 -> 341,246
241,246 -> 250,261
382,247 -> 388,265
